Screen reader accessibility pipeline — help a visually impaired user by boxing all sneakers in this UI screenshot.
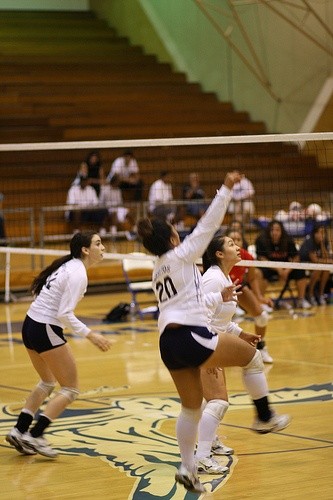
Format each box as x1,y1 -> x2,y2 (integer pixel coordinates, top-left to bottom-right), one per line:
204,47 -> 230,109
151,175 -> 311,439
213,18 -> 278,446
197,458 -> 230,474
5,427 -> 36,455
251,415 -> 292,434
175,468 -> 207,493
20,432 -> 59,458
210,440 -> 234,455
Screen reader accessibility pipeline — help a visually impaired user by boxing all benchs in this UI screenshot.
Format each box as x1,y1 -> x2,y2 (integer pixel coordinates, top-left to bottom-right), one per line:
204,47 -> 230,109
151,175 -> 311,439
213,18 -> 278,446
0,8 -> 333,292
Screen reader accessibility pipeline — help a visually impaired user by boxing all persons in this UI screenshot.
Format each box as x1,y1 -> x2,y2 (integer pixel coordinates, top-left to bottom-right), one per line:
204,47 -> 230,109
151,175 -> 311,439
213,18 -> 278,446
0,147 -> 333,315
136,171 -> 292,494
190,230 -> 263,475
222,227 -> 276,364
5,229 -> 112,460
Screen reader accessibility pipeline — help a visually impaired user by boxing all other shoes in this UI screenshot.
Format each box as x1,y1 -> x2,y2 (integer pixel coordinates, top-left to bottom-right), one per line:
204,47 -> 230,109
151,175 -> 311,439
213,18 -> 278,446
319,296 -> 326,305
296,300 -> 310,308
257,346 -> 273,363
309,297 -> 318,306
261,304 -> 273,312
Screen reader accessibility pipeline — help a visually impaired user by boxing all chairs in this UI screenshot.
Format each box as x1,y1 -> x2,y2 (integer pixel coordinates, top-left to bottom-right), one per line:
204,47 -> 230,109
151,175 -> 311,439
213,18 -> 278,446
121,252 -> 159,320
265,272 -> 305,310
